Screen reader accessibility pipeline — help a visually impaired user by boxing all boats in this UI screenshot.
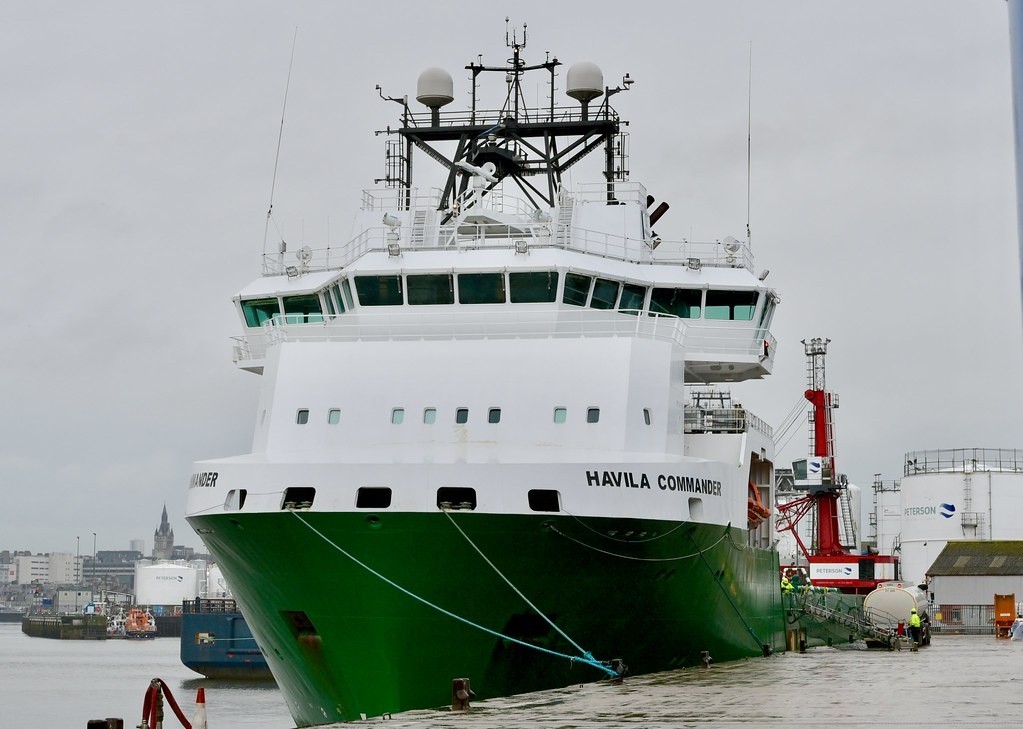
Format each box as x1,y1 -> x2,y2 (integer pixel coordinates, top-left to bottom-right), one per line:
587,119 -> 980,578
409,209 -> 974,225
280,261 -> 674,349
181,592 -> 276,680
22,596 -> 243,639
104,608 -> 161,638
187,17 -> 864,725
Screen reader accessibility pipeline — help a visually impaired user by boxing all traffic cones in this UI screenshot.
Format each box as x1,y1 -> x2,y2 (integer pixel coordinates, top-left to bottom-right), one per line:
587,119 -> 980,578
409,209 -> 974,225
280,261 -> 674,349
190,688 -> 210,728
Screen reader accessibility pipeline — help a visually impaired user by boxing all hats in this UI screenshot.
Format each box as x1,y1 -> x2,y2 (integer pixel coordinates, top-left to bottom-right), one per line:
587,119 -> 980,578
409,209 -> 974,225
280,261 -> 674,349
783,578 -> 788,583
911,608 -> 916,612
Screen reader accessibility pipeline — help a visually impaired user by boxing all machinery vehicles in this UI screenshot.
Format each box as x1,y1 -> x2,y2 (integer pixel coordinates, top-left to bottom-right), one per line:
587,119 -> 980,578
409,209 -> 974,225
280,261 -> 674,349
864,580 -> 934,651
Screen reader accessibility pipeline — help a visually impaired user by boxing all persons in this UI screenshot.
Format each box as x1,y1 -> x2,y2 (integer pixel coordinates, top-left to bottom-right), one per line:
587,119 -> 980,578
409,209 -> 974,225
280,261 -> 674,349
781,579 -> 795,592
41,608 -> 51,616
922,611 -> 931,627
908,608 -> 920,647
